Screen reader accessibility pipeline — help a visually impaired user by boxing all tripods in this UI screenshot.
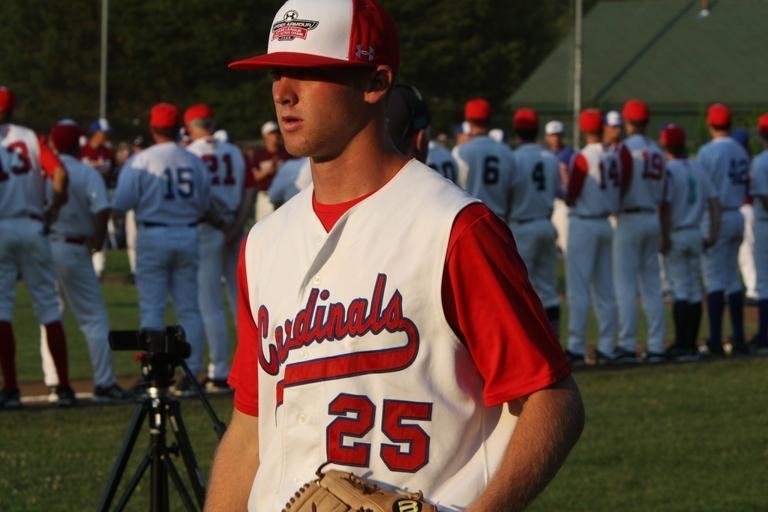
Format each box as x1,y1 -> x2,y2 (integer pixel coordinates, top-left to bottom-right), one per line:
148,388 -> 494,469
95,379 -> 206,512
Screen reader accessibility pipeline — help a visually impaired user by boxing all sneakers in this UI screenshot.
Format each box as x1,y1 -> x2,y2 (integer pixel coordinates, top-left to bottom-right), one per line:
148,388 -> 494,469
204,378 -> 233,394
699,344 -> 725,355
175,376 -> 201,397
48,385 -> 78,406
723,341 -> 748,354
640,351 -> 665,362
567,350 -> 588,365
745,341 -> 767,356
668,345 -> 700,361
589,350 -> 614,362
613,349 -> 638,363
0,388 -> 20,406
95,382 -> 136,403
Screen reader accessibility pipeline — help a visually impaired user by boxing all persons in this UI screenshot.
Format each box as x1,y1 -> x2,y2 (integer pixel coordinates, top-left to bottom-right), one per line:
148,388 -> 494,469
0,86 -> 80,410
696,103 -> 752,358
246,122 -> 292,224
506,108 -> 570,351
606,113 -> 624,146
451,99 -> 515,222
116,142 -> 133,171
426,128 -> 459,183
541,121 -> 580,253
376,82 -> 430,164
746,118 -> 768,355
79,128 -> 115,171
111,103 -> 213,399
609,99 -> 673,366
204,1 -> 586,512
656,122 -> 709,363
565,107 -> 617,365
183,102 -> 245,395
40,119 -> 137,405
488,108 -> 515,139
269,152 -> 307,206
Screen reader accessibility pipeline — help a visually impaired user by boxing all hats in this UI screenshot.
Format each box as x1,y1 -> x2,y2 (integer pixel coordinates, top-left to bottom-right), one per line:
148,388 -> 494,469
459,122 -> 475,135
545,122 -> 566,135
48,120 -> 89,149
731,129 -> 748,144
214,130 -> 232,142
513,108 -> 539,131
0,87 -> 15,113
490,129 -> 506,140
605,112 -> 623,128
580,109 -> 603,131
465,98 -> 490,119
90,117 -> 115,134
758,113 -> 768,134
183,104 -> 215,136
624,99 -> 650,122
388,84 -> 433,145
662,123 -> 688,146
229,0 -> 402,73
707,104 -> 731,126
263,121 -> 279,136
150,104 -> 181,130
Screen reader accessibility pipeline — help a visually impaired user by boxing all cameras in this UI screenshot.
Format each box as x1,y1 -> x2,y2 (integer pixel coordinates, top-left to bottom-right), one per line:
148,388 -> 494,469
107,323 -> 192,379
